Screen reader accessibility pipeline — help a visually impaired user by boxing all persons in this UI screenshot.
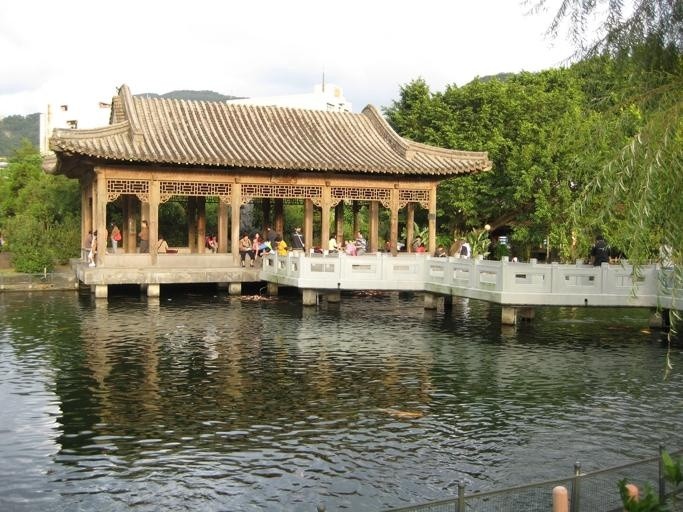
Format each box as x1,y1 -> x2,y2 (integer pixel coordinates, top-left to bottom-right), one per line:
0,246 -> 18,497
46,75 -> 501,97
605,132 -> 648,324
657,237 -> 674,288
328,233 -> 338,253
110,220 -> 120,255
86,231 -> 93,248
238,232 -> 255,268
291,225 -> 305,250
205,234 -> 218,253
351,230 -> 366,256
383,240 -> 391,251
250,225 -> 279,258
399,243 -> 406,251
343,240 -> 356,255
87,230 -> 97,267
137,220 -> 149,253
412,235 -> 423,252
415,243 -> 425,252
157,234 -> 168,254
273,236 -> 290,256
434,244 -> 447,256
589,235 -> 610,267
